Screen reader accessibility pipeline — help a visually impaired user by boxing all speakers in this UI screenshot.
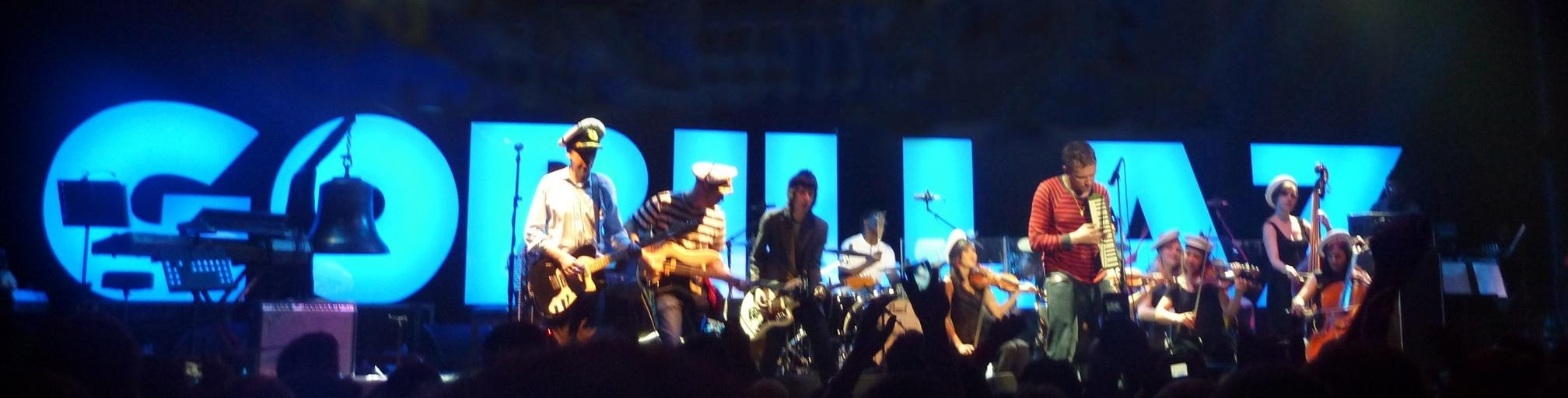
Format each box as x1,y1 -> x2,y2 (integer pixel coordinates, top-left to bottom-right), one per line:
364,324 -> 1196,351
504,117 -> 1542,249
355,303 -> 434,375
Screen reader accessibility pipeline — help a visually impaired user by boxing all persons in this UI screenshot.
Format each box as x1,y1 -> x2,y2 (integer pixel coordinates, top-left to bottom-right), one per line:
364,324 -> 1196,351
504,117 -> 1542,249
942,239 -> 1023,357
838,202 -> 895,306
1264,176 -> 1330,330
625,160 -> 735,348
1155,235 -> 1247,372
750,168 -> 831,383
1136,230 -> 1243,327
1288,237 -> 1371,318
1025,139 -> 1116,364
523,116 -> 642,345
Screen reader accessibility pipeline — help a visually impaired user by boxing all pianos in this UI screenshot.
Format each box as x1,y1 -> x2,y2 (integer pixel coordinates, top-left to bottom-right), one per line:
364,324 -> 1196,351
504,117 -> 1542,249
178,209 -> 295,233
94,229 -> 262,256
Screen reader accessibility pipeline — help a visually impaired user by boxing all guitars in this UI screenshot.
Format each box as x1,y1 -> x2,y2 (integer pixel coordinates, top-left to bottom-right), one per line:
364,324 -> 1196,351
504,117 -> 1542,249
526,217 -> 698,319
740,252 -> 876,342
637,236 -> 802,294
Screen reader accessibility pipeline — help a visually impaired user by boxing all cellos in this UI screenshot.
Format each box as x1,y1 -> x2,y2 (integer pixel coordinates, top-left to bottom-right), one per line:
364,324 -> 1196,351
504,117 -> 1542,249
1303,234 -> 1368,364
1291,162 -> 1327,298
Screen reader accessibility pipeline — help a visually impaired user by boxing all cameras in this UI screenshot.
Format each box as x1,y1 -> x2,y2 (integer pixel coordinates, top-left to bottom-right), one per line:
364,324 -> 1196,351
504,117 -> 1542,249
905,261 -> 933,292
699,316 -> 728,339
1169,361 -> 1192,379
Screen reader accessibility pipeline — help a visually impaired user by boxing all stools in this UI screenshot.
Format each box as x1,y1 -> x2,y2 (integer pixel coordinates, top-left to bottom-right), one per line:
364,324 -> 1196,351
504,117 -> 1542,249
98,270 -> 155,341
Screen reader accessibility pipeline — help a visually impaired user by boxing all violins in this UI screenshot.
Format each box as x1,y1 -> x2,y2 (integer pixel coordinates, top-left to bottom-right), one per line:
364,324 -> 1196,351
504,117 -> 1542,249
1125,266 -> 1165,288
969,265 -> 1049,303
1194,258 -> 1260,289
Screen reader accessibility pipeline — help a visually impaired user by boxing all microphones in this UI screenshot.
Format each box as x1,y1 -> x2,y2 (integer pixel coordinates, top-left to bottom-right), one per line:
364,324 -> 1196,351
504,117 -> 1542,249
514,143 -> 523,150
1108,158 -> 1123,184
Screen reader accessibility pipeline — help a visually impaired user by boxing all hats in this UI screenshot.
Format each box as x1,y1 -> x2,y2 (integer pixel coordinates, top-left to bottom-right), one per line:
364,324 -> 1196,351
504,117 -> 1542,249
1150,229 -> 1180,251
691,159 -> 738,196
1264,173 -> 1297,209
1186,234 -> 1214,256
1317,227 -> 1353,260
558,117 -> 607,153
945,227 -> 970,266
